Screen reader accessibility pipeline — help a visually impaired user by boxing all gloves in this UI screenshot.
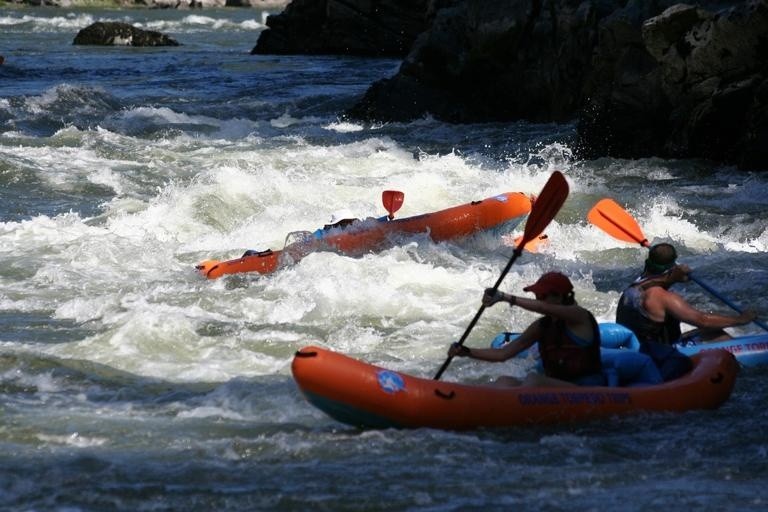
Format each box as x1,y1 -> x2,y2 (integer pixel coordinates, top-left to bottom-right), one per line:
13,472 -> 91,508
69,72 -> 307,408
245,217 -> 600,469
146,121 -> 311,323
448,343 -> 469,358
483,288 -> 504,306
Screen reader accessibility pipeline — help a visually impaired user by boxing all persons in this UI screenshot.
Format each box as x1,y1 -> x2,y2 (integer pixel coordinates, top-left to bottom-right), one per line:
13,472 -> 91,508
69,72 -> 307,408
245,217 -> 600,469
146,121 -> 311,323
447,270 -> 601,386
614,243 -> 759,378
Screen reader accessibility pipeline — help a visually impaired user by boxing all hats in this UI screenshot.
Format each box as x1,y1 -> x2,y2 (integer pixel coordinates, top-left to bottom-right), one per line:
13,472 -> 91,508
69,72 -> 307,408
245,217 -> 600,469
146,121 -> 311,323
524,273 -> 573,299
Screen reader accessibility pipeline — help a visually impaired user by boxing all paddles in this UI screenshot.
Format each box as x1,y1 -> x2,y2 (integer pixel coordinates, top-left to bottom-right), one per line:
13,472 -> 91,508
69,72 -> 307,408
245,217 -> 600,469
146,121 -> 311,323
587,199 -> 768,333
380,190 -> 405,220
433,171 -> 569,381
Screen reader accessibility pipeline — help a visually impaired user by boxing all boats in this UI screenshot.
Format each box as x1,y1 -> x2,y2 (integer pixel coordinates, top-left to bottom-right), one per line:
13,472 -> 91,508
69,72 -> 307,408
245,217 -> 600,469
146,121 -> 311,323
194,191 -> 538,282
488,319 -> 763,378
289,343 -> 735,431
